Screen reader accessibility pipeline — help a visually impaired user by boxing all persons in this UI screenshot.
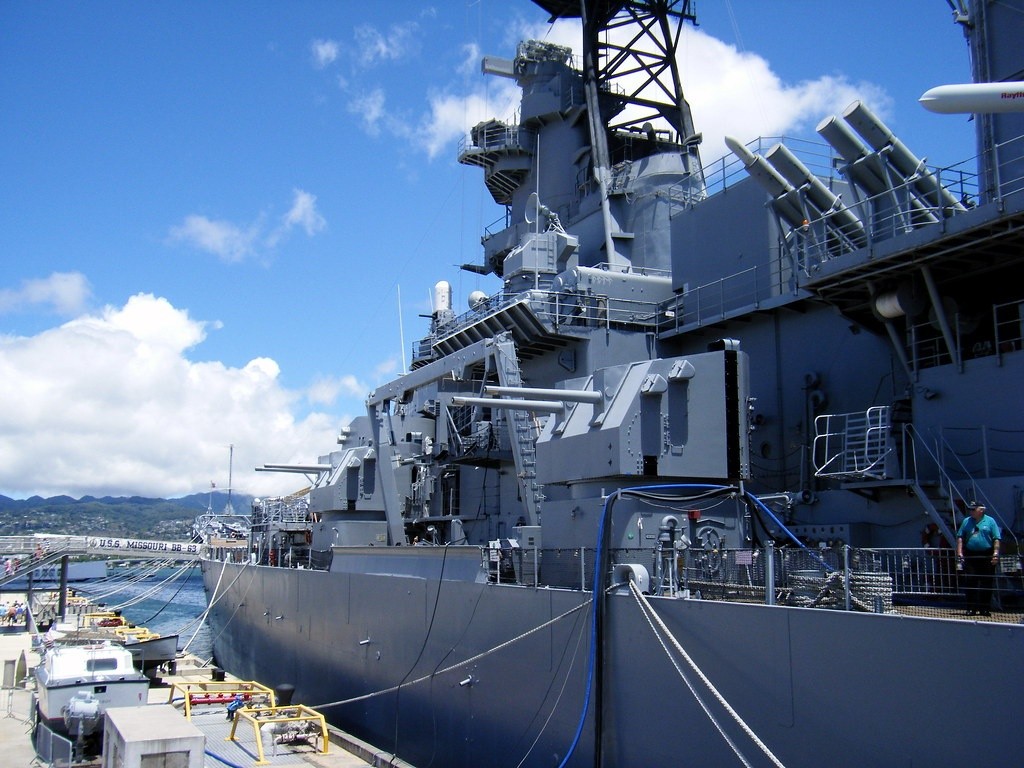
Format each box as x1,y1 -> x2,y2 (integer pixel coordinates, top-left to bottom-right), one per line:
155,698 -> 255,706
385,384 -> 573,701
226,693 -> 244,722
13,558 -> 21,573
0,599 -> 27,626
34,538 -> 49,561
3,557 -> 11,577
955,500 -> 1001,618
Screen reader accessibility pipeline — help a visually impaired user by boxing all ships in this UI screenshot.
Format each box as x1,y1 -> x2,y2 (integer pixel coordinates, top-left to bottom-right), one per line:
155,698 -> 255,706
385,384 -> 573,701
198,0 -> 1022,768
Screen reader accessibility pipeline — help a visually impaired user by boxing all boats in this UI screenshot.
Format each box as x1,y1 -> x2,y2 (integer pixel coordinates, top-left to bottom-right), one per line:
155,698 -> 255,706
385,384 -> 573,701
106,556 -> 159,582
127,634 -> 181,670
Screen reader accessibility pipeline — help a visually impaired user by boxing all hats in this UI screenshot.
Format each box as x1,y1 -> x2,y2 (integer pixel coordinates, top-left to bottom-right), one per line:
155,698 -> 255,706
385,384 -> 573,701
968,500 -> 984,508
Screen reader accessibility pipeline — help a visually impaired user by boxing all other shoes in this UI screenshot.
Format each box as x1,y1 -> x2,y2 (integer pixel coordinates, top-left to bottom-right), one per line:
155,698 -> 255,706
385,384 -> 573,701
980,610 -> 991,616
966,608 -> 976,616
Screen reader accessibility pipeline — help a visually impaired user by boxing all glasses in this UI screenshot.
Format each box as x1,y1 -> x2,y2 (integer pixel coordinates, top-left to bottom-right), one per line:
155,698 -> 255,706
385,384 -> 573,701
970,507 -> 975,511
